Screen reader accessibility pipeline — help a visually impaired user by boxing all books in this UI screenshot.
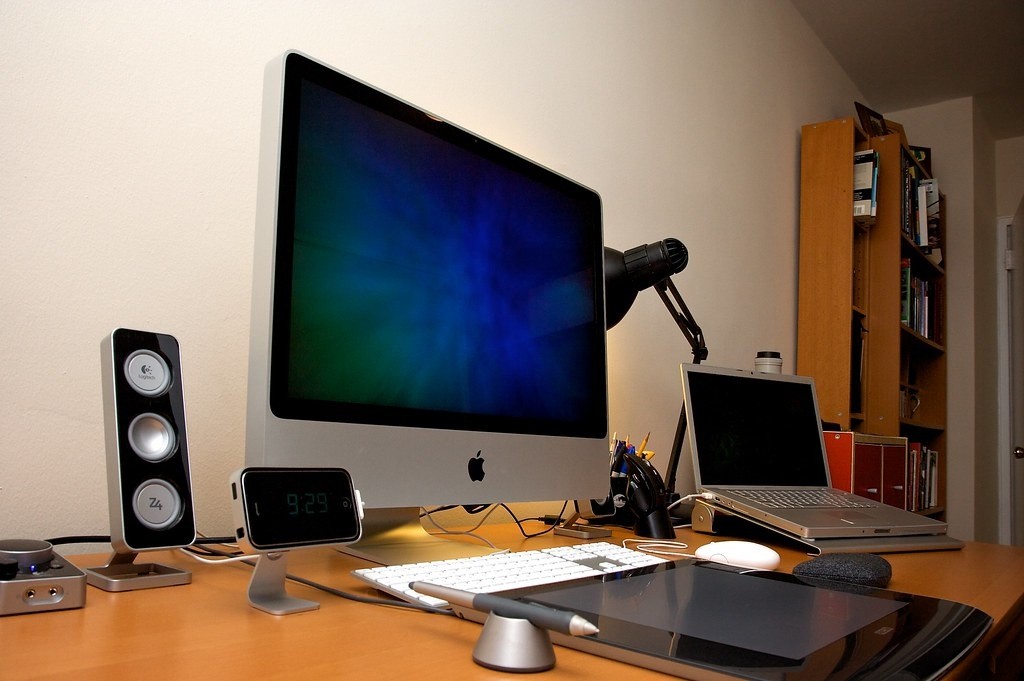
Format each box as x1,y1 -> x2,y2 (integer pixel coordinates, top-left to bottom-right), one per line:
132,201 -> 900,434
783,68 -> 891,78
901,258 -> 929,339
901,151 -> 941,249
908,443 -> 939,511
853,149 -> 879,225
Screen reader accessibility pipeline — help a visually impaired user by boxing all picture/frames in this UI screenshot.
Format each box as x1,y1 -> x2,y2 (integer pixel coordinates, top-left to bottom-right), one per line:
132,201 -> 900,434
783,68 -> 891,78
855,101 -> 931,176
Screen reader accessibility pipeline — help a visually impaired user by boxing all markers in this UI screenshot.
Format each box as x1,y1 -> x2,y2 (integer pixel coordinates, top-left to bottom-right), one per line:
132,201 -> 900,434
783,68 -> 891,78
611,444 -> 636,478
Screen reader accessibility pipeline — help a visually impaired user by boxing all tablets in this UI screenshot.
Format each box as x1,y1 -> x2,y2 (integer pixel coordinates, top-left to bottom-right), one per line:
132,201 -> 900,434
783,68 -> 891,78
449,556 -> 993,681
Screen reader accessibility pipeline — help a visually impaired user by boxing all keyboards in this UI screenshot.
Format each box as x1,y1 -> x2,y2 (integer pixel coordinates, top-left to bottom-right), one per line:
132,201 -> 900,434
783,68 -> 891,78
350,541 -> 667,610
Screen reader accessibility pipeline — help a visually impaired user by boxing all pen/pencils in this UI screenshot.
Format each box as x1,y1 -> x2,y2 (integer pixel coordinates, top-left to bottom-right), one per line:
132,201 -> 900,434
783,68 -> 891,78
609,431 -> 656,461
409,579 -> 601,637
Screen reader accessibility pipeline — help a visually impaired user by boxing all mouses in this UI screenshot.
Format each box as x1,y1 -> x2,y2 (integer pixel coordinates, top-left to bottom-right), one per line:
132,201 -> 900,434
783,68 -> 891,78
695,540 -> 779,571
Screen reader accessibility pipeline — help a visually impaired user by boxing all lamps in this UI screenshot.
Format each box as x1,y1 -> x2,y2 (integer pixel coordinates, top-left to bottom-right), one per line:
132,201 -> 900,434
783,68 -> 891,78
603,236 -> 708,527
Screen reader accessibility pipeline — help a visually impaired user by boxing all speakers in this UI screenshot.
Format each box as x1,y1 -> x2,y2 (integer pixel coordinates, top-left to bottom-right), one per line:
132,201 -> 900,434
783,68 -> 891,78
78,327 -> 198,592
554,487 -> 617,538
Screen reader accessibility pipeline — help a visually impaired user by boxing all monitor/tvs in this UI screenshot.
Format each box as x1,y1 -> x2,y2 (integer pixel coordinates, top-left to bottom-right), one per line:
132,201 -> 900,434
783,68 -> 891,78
239,49 -> 611,558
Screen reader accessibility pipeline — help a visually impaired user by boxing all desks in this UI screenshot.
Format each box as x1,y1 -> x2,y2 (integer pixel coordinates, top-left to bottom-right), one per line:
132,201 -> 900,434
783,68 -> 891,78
0,498 -> 1024,681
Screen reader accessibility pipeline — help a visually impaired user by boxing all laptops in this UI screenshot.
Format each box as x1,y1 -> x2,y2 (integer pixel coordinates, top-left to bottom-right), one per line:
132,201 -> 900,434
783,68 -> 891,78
680,362 -> 950,537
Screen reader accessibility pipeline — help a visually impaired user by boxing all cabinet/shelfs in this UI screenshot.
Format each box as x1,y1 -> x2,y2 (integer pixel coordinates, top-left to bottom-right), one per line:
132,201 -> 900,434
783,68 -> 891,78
795,116 -> 949,536
822,430 -> 908,513
